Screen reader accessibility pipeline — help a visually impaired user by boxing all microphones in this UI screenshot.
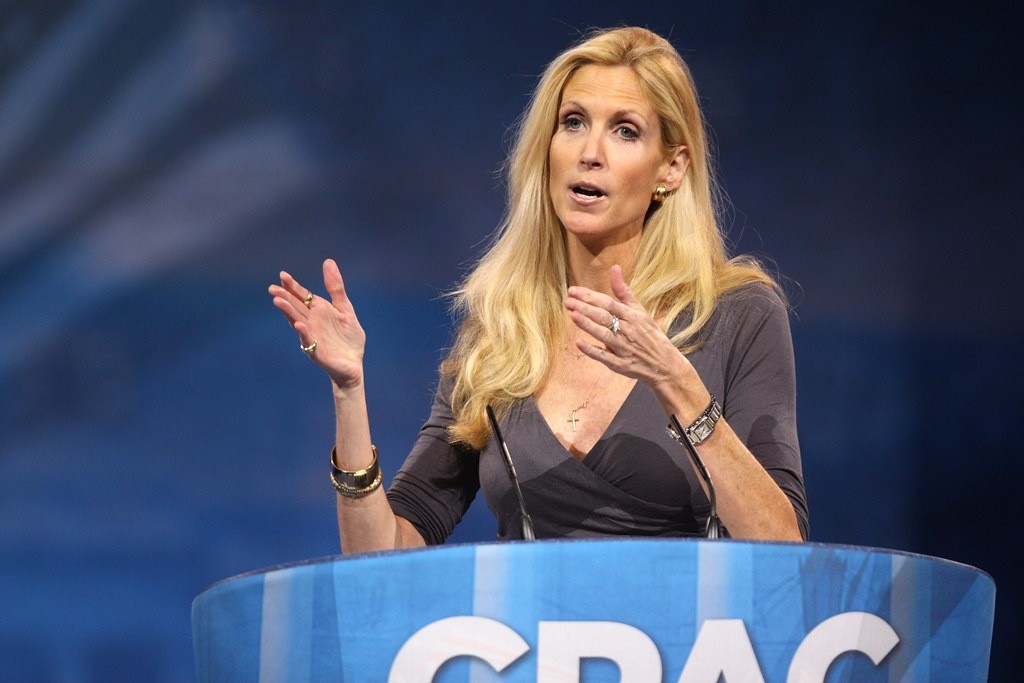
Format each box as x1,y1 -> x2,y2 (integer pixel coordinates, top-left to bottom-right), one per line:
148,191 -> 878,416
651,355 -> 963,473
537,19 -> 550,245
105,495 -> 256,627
487,405 -> 536,541
670,413 -> 721,540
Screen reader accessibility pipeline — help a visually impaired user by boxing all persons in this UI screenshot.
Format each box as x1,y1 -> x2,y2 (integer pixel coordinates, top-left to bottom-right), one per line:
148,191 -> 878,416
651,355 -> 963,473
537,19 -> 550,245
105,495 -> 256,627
268,27 -> 809,553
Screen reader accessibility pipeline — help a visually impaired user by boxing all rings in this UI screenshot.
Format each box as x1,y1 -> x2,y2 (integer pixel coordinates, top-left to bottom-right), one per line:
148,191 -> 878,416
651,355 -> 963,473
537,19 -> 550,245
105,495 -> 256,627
301,340 -> 317,354
607,315 -> 621,334
302,290 -> 313,306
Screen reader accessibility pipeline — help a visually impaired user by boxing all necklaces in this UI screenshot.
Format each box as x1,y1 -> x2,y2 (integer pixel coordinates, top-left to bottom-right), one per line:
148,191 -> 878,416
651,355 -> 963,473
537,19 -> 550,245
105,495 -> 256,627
559,344 -> 605,432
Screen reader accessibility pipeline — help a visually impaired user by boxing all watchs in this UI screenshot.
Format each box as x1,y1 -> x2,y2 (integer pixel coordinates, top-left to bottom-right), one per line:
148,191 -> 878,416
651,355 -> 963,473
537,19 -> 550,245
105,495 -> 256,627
665,394 -> 721,446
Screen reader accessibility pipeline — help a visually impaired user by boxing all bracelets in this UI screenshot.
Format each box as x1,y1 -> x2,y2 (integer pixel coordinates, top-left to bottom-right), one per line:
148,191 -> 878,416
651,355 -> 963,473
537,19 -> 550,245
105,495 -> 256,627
330,445 -> 383,499
